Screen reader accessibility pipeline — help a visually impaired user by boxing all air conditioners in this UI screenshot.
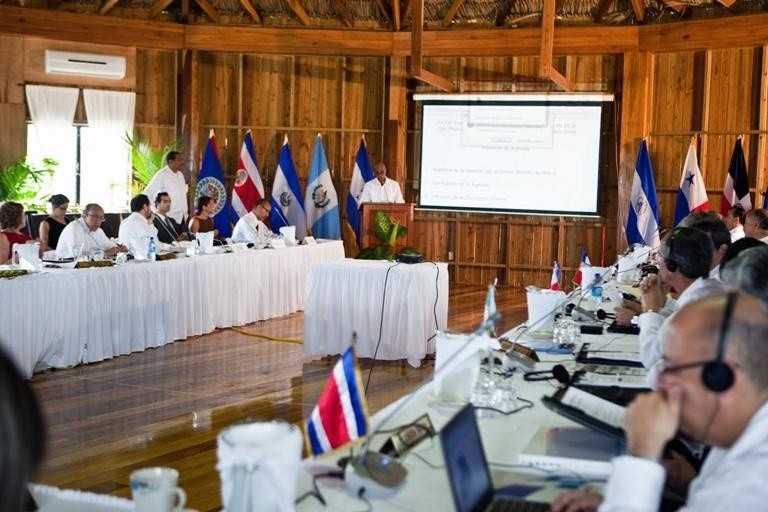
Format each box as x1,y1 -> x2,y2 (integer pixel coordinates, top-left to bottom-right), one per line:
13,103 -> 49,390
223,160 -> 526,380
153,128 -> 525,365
44,49 -> 126,80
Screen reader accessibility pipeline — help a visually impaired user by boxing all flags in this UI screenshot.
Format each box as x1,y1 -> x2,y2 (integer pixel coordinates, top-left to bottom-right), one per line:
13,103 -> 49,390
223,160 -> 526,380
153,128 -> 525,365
479,279 -> 498,347
717,138 -> 753,225
194,130 -> 235,241
623,136 -> 662,252
346,133 -> 376,241
301,132 -> 343,242
270,132 -> 310,243
671,139 -> 709,234
297,338 -> 372,458
229,129 -> 264,230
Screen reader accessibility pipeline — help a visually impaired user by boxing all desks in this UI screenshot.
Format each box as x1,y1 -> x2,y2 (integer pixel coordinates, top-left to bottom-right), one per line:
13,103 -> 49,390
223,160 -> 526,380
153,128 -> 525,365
0,234 -> 348,383
225,265 -> 691,512
309,259 -> 449,372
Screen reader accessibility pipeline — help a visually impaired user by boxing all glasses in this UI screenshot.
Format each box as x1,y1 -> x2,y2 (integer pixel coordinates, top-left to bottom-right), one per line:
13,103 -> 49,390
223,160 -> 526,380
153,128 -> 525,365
52,206 -> 68,210
86,213 -> 106,222
261,204 -> 271,214
651,357 -> 725,380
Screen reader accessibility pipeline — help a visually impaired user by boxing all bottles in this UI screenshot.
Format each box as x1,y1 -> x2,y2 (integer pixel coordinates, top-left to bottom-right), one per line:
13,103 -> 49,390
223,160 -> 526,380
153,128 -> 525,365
146,236 -> 156,261
590,273 -> 605,309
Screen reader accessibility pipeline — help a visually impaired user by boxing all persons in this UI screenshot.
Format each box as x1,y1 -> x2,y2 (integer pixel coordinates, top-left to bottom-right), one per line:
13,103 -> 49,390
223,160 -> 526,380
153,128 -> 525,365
152,191 -> 197,253
0,344 -> 56,512
38,190 -> 72,250
358,162 -> 405,208
117,192 -> 171,259
0,201 -> 49,267
141,148 -> 192,233
228,197 -> 280,243
53,202 -> 128,261
187,195 -> 226,247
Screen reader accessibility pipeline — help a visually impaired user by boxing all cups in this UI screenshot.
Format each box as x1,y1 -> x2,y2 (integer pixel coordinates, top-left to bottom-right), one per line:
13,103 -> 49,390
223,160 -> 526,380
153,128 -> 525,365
129,466 -> 187,511
42,246 -> 128,266
471,372 -> 518,411
552,321 -> 581,348
215,418 -> 302,511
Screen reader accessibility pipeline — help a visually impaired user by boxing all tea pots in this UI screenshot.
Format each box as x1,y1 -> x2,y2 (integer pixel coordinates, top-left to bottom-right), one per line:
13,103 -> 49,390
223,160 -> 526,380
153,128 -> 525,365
432,326 -> 492,404
11,244 -> 40,273
185,226 -> 297,256
577,265 -> 612,300
524,284 -> 566,338
616,243 -> 649,285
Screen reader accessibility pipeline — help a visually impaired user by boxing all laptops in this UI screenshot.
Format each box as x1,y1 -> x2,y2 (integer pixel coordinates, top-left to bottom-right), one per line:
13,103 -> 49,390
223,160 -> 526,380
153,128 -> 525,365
439,402 -> 583,512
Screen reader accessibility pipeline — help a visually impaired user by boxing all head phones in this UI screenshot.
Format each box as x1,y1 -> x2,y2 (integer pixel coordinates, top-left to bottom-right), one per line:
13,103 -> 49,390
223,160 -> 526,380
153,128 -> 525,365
664,227 -> 693,272
622,292 -> 642,306
553,303 -> 595,322
702,293 -> 737,392
523,364 -> 570,384
597,309 -> 616,320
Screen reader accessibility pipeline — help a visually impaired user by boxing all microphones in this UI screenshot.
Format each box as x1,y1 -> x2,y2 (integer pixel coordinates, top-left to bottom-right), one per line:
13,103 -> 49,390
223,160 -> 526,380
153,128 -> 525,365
344,311 -> 504,500
382,180 -> 389,201
505,226 -> 675,367
275,208 -> 303,244
190,231 -> 232,253
236,211 -> 265,248
72,215 -> 113,262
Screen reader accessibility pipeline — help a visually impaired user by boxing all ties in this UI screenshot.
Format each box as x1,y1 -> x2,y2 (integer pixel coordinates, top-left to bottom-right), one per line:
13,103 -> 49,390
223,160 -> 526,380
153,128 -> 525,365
164,217 -> 171,228
